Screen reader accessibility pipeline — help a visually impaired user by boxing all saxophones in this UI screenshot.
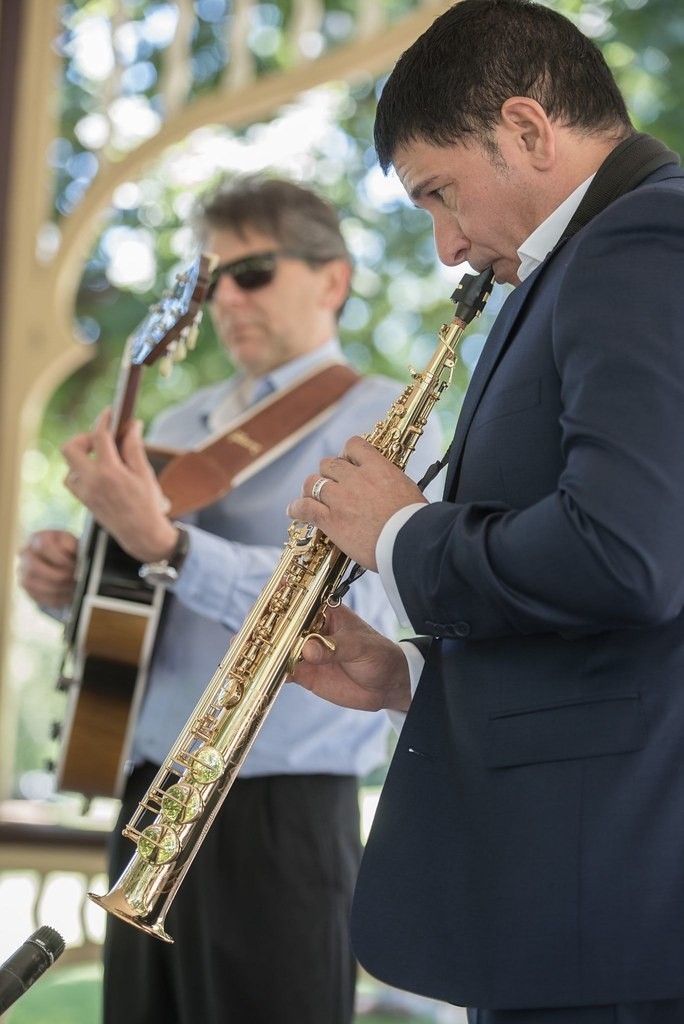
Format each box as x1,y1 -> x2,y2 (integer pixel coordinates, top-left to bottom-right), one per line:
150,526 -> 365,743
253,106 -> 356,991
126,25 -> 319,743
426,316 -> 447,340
86,267 -> 499,943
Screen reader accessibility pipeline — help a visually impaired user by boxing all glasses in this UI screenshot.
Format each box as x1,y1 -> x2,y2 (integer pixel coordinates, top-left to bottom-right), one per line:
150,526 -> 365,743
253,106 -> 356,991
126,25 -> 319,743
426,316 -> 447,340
203,250 -> 327,303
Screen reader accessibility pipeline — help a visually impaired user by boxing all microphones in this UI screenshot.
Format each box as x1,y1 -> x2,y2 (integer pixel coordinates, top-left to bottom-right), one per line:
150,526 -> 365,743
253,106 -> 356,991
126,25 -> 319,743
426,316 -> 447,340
0,926 -> 67,1015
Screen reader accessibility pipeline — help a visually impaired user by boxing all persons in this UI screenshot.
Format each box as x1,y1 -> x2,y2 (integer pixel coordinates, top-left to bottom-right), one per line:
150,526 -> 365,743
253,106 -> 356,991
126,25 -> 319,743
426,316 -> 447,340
287,0 -> 684,1024
19,175 -> 449,1024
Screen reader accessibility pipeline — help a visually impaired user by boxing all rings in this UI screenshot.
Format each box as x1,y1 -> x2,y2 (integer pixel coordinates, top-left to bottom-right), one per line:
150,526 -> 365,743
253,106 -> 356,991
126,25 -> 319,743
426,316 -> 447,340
312,477 -> 333,500
72,475 -> 79,485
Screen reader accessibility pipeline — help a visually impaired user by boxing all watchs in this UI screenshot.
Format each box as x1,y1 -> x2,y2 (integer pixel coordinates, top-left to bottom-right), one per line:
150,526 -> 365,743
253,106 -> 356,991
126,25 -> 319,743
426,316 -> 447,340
139,527 -> 189,587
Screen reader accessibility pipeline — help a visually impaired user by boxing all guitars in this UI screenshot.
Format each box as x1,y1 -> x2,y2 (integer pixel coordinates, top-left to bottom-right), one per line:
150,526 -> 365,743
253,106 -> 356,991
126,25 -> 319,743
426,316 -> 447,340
39,253 -> 216,800
